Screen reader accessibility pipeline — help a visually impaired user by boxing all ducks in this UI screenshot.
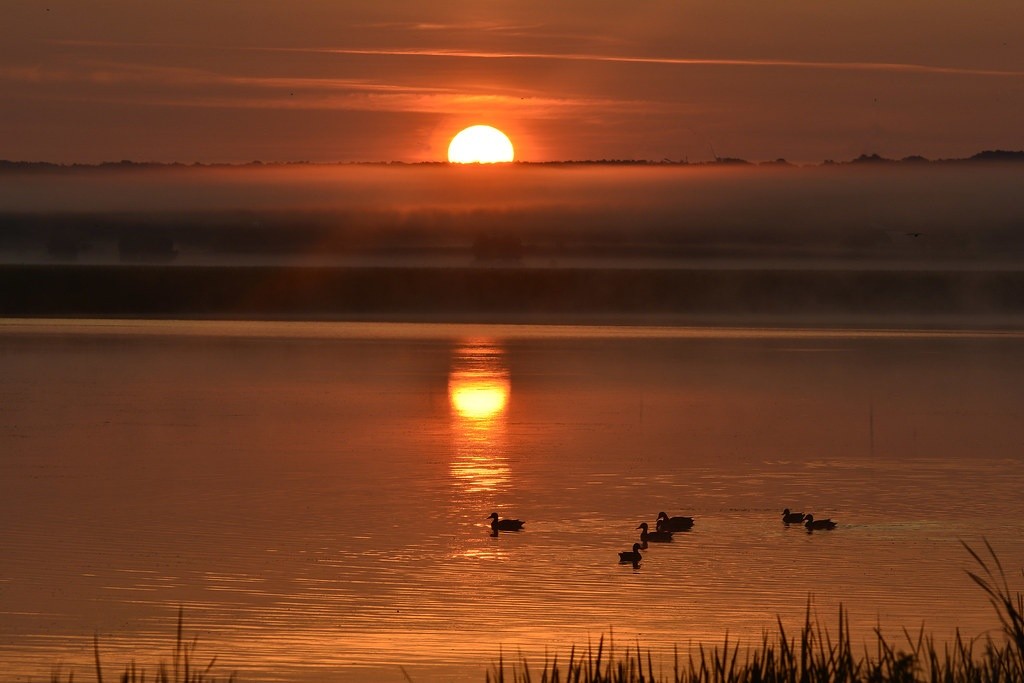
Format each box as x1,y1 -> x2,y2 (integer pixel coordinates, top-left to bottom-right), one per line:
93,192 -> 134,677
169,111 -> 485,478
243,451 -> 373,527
637,523 -> 673,541
655,512 -> 695,531
618,543 -> 642,562
486,512 -> 526,531
781,508 -> 838,530
